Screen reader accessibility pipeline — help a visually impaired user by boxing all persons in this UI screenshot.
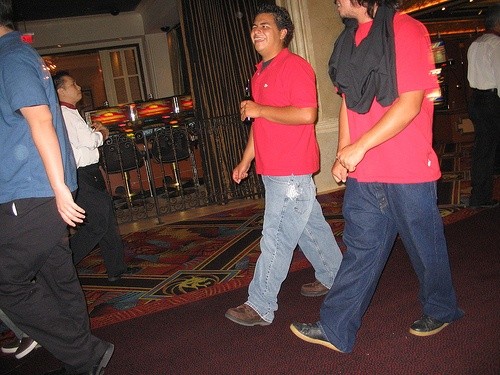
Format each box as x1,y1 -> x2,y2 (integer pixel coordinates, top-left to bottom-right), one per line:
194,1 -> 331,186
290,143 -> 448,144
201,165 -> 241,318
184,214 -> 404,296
289,0 -> 465,353
0,0 -> 115,375
465,9 -> 499,208
0,309 -> 38,360
225,5 -> 343,327
50,70 -> 142,282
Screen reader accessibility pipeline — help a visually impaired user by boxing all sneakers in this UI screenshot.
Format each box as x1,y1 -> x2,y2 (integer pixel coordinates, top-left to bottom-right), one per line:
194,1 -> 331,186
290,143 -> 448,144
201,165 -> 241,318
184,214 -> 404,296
15,337 -> 38,359
409,314 -> 454,336
1,337 -> 21,353
225,303 -> 270,326
290,322 -> 345,353
301,280 -> 329,296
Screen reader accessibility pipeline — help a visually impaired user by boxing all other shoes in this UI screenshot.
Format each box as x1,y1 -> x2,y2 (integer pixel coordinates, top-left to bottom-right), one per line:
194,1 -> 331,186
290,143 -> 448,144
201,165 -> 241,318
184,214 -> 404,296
471,199 -> 499,209
81,342 -> 114,375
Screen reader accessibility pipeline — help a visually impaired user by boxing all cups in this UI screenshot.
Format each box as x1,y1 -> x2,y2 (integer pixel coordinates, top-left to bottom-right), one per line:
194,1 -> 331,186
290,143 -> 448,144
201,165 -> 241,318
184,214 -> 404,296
172,97 -> 180,113
130,106 -> 138,121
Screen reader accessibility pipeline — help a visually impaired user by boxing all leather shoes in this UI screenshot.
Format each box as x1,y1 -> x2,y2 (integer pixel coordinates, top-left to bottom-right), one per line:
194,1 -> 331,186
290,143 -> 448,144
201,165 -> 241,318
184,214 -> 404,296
108,267 -> 142,281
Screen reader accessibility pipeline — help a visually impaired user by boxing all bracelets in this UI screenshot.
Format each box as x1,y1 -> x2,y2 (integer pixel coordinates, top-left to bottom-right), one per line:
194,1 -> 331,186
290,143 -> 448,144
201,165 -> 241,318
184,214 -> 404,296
99,129 -> 107,142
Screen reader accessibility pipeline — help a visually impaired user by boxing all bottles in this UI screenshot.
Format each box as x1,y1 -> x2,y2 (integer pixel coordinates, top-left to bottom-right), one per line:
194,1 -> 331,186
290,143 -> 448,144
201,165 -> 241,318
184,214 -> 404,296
243,80 -> 253,124
